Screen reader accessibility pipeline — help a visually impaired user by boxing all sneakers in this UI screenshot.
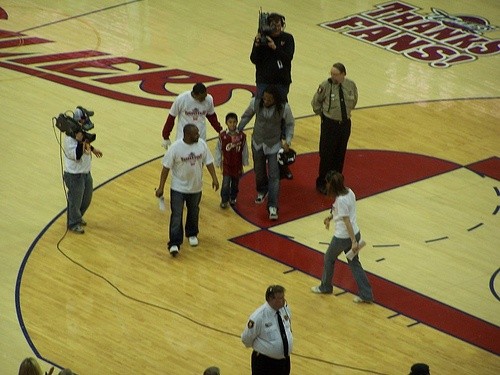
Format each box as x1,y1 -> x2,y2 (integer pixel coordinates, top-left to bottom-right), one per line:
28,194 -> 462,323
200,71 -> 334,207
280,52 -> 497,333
170,245 -> 178,256
220,202 -> 228,208
317,186 -> 328,195
81,219 -> 86,226
269,206 -> 278,220
352,296 -> 374,303
229,198 -> 237,205
254,190 -> 269,204
70,224 -> 85,234
312,285 -> 332,293
188,235 -> 199,246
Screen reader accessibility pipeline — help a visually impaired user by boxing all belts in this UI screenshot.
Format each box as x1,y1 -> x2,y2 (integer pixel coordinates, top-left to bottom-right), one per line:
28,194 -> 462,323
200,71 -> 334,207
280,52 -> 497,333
325,115 -> 350,124
256,351 -> 290,362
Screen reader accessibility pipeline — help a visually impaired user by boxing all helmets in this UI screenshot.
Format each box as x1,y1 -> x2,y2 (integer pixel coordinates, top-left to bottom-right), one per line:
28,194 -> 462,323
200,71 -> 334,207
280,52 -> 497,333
277,148 -> 296,166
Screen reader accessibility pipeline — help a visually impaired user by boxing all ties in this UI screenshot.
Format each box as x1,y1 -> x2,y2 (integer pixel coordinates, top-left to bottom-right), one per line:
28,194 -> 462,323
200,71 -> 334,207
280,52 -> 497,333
339,82 -> 347,123
277,311 -> 289,359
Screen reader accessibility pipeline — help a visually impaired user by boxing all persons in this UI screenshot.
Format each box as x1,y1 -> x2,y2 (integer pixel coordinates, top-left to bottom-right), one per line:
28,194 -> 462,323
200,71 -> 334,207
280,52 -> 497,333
310,62 -> 358,196
18,357 -> 54,375
408,363 -> 430,375
248,12 -> 295,141
214,112 -> 250,209
158,82 -> 224,169
61,105 -> 104,235
154,124 -> 220,256
236,83 -> 295,221
310,168 -> 375,304
240,284 -> 295,375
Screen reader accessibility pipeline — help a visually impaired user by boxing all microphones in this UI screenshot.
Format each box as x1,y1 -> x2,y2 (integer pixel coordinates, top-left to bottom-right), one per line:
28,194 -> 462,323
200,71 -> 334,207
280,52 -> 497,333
285,300 -> 287,307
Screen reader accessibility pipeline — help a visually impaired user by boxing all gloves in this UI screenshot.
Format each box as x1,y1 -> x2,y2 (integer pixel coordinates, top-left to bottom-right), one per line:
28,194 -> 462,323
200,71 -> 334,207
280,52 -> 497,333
161,139 -> 171,149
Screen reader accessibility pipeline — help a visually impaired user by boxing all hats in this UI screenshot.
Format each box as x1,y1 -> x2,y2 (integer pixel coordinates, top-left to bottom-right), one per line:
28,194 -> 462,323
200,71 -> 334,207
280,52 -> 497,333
73,106 -> 94,120
